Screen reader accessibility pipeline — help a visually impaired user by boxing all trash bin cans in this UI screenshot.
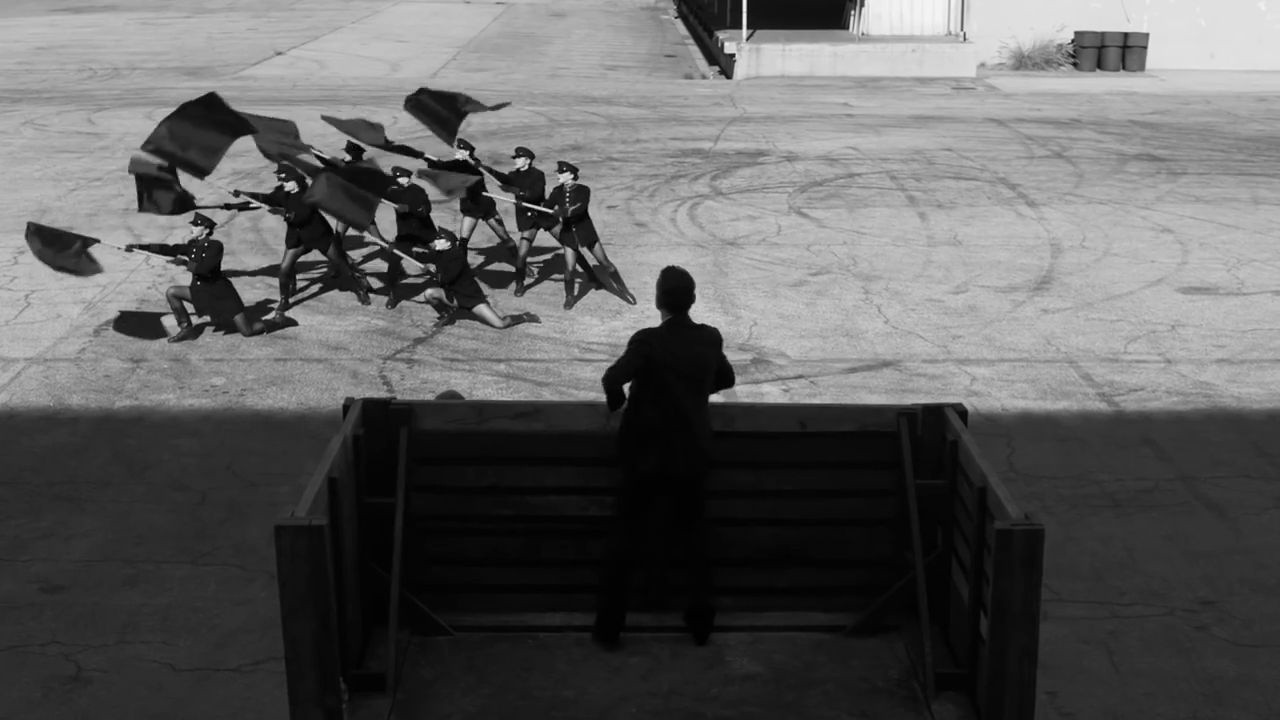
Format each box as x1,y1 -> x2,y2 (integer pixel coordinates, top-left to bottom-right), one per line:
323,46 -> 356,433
1074,31 -> 1101,72
1098,31 -> 1125,71
1123,32 -> 1150,71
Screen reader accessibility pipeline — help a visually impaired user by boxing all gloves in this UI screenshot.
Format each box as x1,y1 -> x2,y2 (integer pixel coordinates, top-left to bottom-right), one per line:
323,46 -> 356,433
126,244 -> 139,253
166,254 -> 186,266
268,207 -> 287,217
234,189 -> 247,198
386,181 -> 570,253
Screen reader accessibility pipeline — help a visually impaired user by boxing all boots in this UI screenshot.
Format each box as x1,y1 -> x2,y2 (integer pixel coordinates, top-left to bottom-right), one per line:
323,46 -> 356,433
275,280 -> 290,311
289,270 -> 296,298
432,302 -> 457,328
458,236 -> 467,258
514,265 -> 525,297
508,245 -> 537,277
577,256 -> 605,289
167,305 -> 196,344
387,280 -> 399,310
261,311 -> 299,333
502,312 -> 542,326
347,272 -> 370,304
608,269 -> 637,305
564,270 -> 573,310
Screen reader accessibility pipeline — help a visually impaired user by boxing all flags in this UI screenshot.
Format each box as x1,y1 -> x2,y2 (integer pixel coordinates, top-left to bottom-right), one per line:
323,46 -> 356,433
142,92 -> 256,179
128,155 -> 198,215
25,221 -> 103,276
301,169 -> 380,234
417,167 -> 484,200
404,87 -> 512,148
320,114 -> 423,159
234,110 -> 311,159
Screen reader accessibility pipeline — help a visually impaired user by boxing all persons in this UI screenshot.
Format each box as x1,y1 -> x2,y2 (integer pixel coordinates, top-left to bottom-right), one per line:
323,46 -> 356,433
227,139 -> 438,308
417,139 -> 520,256
126,211 -> 299,344
592,265 -> 734,650
389,224 -> 542,327
517,161 -> 638,310
468,145 -> 599,297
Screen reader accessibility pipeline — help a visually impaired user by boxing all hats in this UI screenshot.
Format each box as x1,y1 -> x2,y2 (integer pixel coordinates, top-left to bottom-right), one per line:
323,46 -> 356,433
511,146 -> 536,161
343,140 -> 367,155
390,166 -> 414,180
457,137 -> 475,153
555,161 -> 579,175
282,169 -> 307,185
188,212 -> 217,230
273,163 -> 295,176
